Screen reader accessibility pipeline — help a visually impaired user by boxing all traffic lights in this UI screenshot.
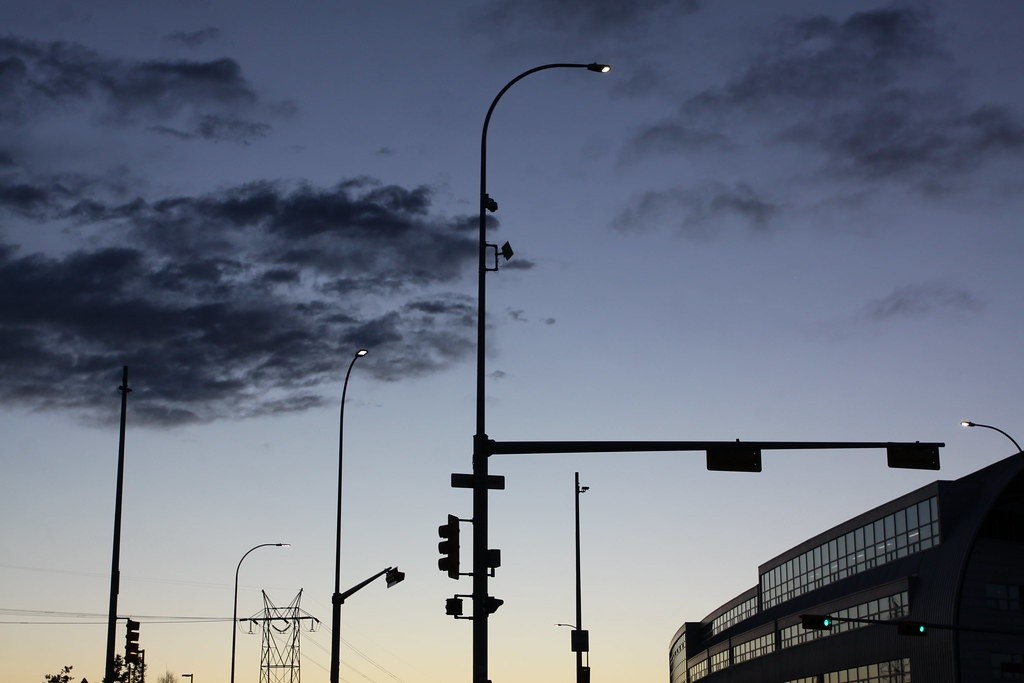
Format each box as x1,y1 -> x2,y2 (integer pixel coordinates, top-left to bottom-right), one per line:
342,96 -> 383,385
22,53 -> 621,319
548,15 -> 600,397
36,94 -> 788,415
898,621 -> 929,636
801,614 -> 833,631
126,618 -> 140,664
438,514 -> 459,580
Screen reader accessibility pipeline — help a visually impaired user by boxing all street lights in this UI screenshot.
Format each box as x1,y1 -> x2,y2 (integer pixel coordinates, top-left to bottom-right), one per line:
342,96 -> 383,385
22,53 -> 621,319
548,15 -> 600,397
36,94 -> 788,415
181,674 -> 193,683
231,543 -> 290,683
960,420 -> 1022,452
474,62 -> 610,683
329,348 -> 406,683
